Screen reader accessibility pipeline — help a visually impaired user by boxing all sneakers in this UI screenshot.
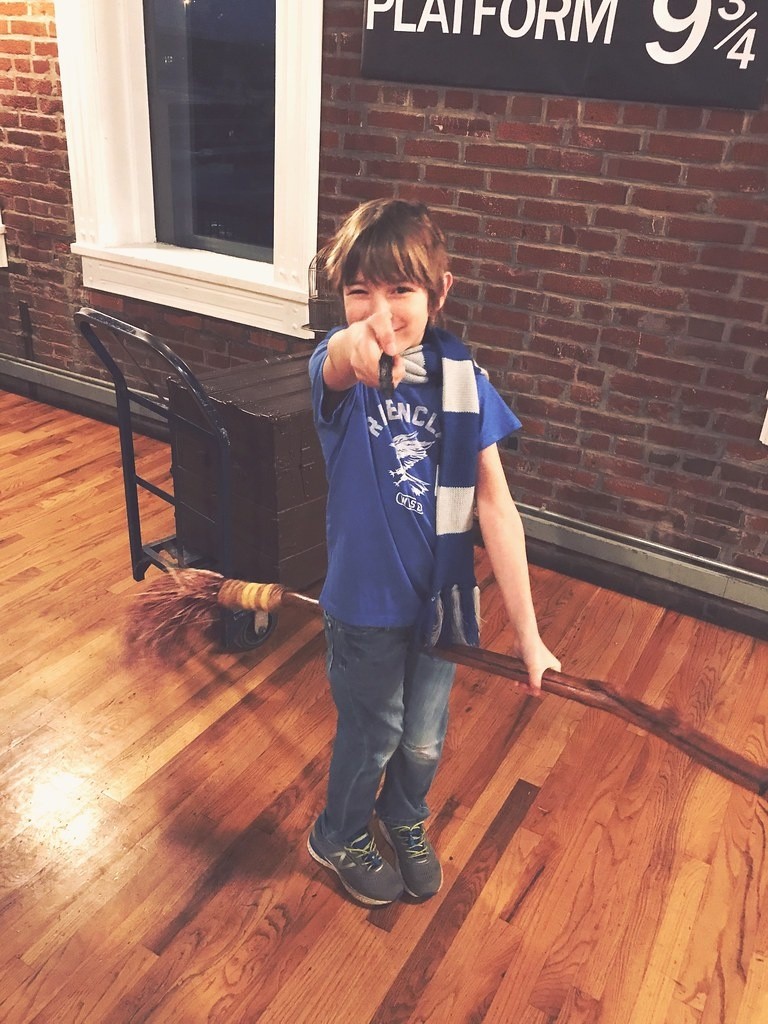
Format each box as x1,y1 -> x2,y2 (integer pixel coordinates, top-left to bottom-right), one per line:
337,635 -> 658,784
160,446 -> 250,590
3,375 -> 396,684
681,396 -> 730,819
377,817 -> 444,898
306,819 -> 404,905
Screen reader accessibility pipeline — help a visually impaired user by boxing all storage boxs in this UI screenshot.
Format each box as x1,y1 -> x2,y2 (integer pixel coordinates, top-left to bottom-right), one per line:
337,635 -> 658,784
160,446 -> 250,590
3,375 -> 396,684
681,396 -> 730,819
166,349 -> 329,592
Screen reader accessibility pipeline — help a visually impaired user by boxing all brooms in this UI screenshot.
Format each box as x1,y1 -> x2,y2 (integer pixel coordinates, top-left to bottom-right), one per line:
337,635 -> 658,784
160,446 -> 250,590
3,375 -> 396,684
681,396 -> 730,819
115,568 -> 767,800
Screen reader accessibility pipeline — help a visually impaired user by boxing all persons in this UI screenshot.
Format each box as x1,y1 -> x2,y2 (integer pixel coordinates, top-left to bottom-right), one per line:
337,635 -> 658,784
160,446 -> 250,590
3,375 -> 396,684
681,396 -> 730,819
303,197 -> 559,906
